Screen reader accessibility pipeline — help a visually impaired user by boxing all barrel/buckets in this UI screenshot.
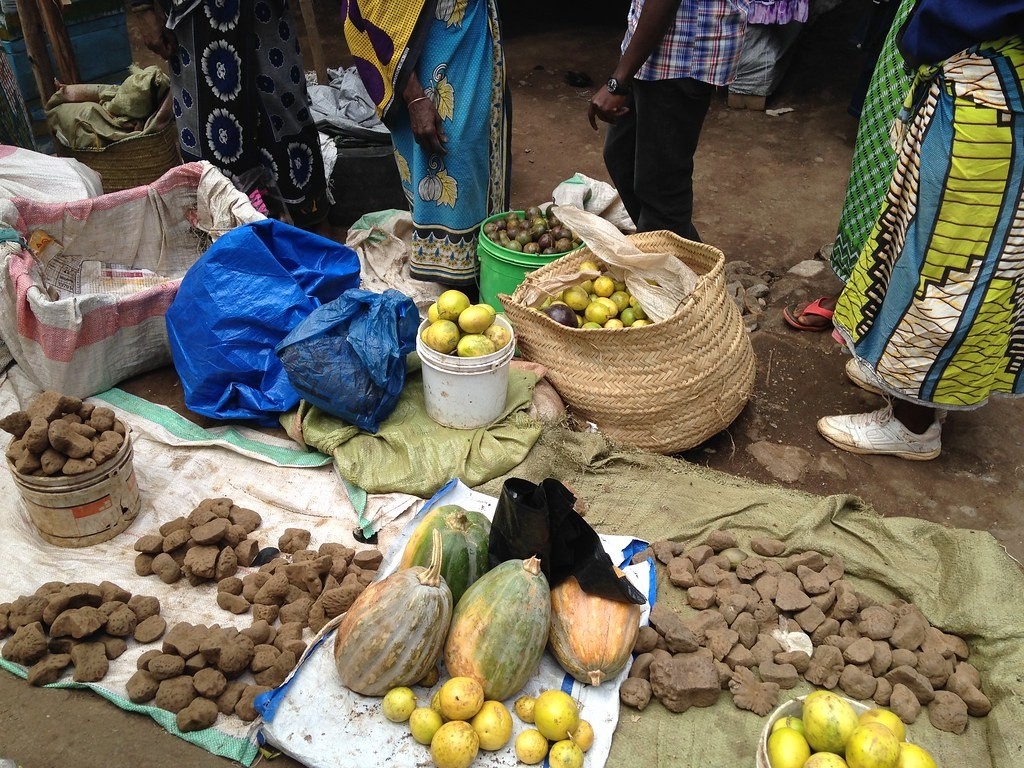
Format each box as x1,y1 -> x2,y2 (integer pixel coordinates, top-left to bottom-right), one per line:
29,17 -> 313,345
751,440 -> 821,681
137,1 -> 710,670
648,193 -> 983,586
477,209 -> 587,356
415,315 -> 513,431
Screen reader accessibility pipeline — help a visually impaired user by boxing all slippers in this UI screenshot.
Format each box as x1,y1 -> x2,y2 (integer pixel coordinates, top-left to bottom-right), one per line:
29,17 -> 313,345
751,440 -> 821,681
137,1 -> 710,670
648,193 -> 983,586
782,296 -> 835,331
831,327 -> 847,347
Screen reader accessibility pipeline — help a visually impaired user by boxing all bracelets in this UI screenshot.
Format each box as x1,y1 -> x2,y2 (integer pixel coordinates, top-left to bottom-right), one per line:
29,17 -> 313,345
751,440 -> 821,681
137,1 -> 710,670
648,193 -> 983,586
404,94 -> 430,108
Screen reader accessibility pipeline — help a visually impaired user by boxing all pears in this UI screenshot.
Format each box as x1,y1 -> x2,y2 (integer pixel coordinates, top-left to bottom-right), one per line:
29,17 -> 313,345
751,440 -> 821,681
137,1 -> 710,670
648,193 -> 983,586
421,290 -> 511,357
524,262 -> 661,329
767,691 -> 936,768
382,676 -> 594,768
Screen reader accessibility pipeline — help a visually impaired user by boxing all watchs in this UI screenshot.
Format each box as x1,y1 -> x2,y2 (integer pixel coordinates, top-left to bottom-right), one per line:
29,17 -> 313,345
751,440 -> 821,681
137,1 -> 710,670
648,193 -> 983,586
606,75 -> 628,96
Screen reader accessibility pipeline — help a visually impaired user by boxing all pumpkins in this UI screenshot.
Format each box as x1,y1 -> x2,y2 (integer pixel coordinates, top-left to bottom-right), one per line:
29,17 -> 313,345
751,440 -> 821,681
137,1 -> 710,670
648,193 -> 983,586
396,504 -> 491,609
334,528 -> 453,697
550,564 -> 640,687
442,554 -> 551,701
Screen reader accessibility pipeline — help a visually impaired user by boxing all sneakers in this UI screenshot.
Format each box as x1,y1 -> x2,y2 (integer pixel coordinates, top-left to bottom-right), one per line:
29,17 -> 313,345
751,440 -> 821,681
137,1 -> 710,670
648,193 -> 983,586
817,404 -> 942,461
845,357 -> 948,419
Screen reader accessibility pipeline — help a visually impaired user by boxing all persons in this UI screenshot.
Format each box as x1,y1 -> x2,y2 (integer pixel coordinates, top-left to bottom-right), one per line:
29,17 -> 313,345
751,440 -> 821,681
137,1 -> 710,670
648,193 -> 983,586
779,0 -> 1023,463
157,0 -> 337,253
340,0 -> 514,304
585,0 -> 747,253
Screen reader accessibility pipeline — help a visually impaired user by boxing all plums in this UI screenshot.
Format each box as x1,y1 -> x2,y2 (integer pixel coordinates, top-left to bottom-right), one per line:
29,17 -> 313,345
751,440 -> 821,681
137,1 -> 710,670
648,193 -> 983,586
483,204 -> 584,254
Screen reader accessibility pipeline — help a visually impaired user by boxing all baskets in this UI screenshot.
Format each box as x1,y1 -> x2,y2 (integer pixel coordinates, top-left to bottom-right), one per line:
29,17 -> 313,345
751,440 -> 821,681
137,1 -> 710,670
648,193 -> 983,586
497,230 -> 757,457
50,118 -> 183,195
